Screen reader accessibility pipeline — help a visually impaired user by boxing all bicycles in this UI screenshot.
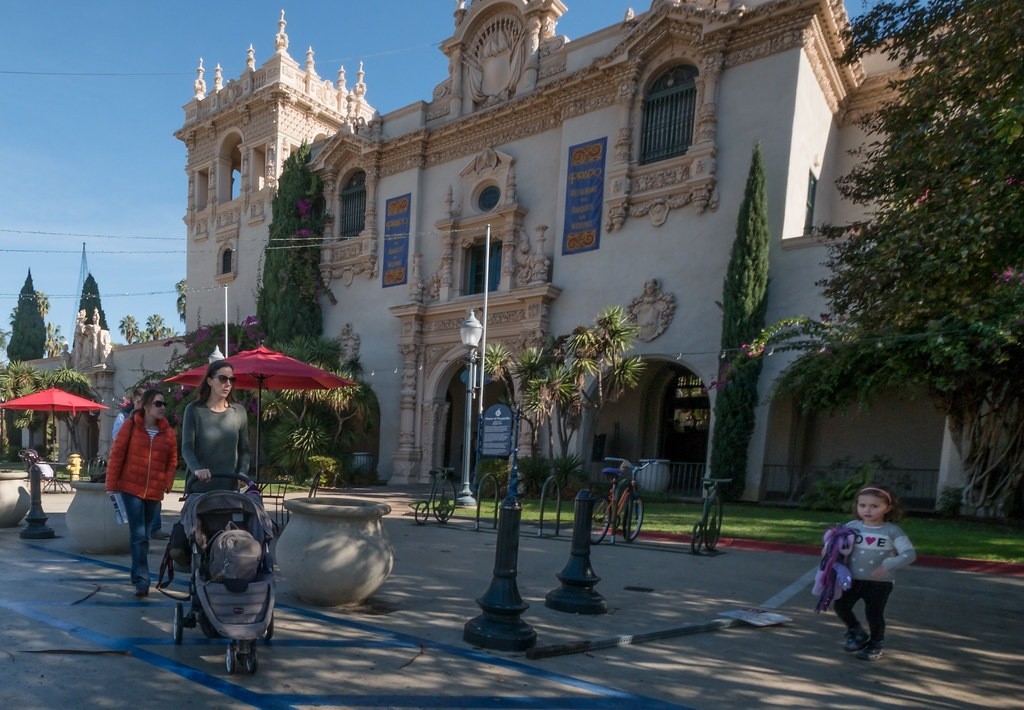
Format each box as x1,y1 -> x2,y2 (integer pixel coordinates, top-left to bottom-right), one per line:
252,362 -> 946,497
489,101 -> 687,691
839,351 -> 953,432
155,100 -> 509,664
690,478 -> 733,555
414,466 -> 456,525
590,458 -> 657,545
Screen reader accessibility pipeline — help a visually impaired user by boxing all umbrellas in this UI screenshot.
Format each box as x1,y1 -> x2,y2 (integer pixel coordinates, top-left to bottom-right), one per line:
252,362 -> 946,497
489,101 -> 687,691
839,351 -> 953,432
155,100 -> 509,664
164,340 -> 357,488
0,387 -> 110,491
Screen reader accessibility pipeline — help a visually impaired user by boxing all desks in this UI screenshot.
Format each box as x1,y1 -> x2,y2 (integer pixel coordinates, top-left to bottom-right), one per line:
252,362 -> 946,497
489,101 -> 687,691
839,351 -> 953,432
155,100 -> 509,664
255,479 -> 288,532
40,461 -> 73,493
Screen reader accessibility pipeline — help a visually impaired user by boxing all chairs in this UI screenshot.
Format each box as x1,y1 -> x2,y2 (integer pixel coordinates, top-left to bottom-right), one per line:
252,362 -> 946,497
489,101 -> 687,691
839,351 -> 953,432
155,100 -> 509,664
19,448 -> 106,489
260,473 -> 322,524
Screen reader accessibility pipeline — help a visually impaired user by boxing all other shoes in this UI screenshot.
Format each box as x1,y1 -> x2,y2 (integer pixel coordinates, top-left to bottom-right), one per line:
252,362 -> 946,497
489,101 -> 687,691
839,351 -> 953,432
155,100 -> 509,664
135,590 -> 149,596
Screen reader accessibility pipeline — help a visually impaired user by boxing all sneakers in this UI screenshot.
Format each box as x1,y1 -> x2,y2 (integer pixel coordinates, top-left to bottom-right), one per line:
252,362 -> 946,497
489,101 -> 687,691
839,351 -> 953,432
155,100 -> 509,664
856,639 -> 884,660
844,628 -> 870,651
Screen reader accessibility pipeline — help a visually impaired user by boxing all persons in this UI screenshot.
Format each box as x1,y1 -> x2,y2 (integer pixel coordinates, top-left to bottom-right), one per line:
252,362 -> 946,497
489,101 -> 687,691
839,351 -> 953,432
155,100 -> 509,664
112,389 -> 170,539
182,361 -> 252,636
821,485 -> 916,660
106,389 -> 177,596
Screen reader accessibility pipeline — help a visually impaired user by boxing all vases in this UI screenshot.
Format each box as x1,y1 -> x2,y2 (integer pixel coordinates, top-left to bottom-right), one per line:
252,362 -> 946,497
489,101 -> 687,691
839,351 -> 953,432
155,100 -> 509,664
639,459 -> 671,494
350,452 -> 374,474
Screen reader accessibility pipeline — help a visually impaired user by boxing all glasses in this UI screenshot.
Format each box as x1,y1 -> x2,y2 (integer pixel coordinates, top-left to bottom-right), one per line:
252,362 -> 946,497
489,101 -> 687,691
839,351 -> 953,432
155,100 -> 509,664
213,375 -> 236,385
152,401 -> 167,407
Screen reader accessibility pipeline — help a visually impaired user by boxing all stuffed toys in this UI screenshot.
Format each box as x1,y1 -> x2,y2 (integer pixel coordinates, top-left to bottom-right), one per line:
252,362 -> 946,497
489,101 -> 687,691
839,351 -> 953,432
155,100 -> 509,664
812,528 -> 856,615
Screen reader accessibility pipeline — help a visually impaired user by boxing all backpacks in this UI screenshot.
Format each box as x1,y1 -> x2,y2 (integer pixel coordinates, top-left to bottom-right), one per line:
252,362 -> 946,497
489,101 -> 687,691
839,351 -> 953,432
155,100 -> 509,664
170,523 -> 192,566
208,521 -> 261,583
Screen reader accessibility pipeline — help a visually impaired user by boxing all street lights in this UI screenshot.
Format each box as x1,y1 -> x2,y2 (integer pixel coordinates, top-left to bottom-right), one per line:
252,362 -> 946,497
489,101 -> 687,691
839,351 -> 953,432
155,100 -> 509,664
457,309 -> 484,506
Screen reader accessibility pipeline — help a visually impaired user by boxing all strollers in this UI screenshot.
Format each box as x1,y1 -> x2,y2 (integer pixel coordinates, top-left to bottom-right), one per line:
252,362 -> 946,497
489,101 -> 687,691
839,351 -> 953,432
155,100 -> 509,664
156,469 -> 275,675
